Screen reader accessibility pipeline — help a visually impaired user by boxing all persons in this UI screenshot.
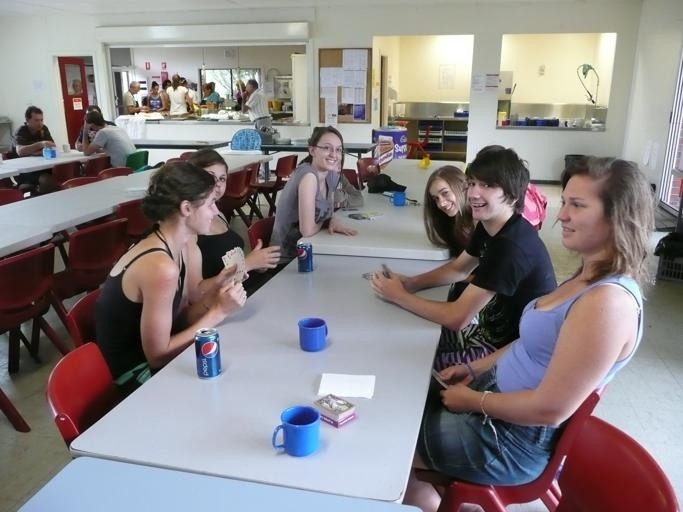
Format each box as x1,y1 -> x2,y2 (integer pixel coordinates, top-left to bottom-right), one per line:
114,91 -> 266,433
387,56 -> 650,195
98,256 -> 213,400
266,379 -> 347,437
122,74 -> 226,117
268,126 -> 358,275
168,147 -> 283,333
72,80 -> 83,95
422,164 -> 478,253
393,156 -> 657,511
5,104 -> 139,195
237,78 -> 277,180
370,146 -> 554,380
90,159 -> 250,407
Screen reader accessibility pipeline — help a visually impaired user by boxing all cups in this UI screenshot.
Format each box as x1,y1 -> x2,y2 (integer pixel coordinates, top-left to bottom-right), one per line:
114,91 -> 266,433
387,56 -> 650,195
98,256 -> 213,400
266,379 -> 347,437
63,144 -> 71,154
389,191 -> 406,206
298,317 -> 328,352
272,404 -> 321,457
225,93 -> 229,100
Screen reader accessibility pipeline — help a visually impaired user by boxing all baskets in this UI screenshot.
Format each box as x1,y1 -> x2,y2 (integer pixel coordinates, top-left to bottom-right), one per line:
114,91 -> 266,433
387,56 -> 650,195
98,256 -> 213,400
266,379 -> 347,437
656,251 -> 683,282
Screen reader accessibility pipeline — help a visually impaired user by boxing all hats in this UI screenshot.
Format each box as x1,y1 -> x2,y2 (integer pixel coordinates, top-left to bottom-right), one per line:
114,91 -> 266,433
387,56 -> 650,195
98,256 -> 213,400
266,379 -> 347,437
367,173 -> 406,193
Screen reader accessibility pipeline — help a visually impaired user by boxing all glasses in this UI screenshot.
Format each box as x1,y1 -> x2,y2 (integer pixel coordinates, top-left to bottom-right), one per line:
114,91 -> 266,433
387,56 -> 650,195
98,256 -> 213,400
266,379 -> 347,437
315,145 -> 344,154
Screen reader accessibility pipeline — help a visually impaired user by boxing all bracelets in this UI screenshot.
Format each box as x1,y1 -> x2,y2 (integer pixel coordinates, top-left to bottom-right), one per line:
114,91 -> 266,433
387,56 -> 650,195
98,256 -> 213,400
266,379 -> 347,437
478,389 -> 495,430
200,295 -> 212,313
462,363 -> 480,382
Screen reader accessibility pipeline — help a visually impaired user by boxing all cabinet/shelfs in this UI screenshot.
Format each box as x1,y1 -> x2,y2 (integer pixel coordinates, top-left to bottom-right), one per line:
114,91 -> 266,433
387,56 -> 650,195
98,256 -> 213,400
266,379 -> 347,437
400,115 -> 468,163
